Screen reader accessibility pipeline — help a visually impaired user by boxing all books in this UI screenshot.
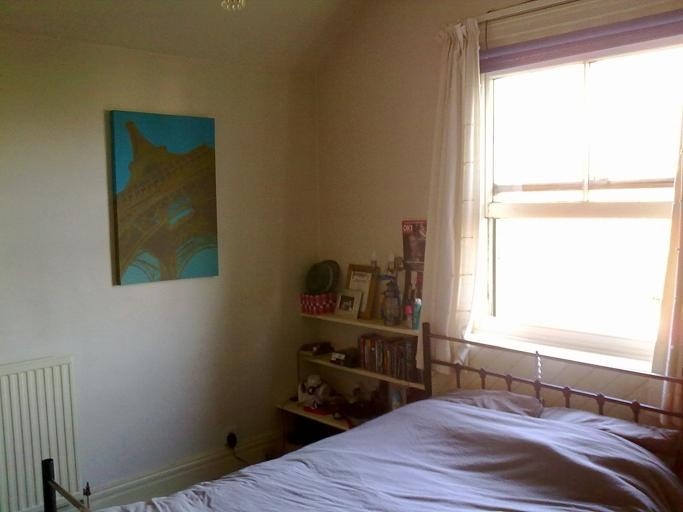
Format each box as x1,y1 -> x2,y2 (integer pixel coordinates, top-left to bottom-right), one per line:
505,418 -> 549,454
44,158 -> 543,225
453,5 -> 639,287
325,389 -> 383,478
358,333 -> 424,385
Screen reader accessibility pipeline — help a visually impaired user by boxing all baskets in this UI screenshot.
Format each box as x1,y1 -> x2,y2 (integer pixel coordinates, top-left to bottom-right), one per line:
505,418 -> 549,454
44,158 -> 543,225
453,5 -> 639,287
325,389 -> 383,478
300,293 -> 337,315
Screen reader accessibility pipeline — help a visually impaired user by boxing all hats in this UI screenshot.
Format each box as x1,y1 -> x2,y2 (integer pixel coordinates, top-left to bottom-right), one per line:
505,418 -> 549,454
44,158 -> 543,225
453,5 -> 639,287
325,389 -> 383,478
306,260 -> 340,294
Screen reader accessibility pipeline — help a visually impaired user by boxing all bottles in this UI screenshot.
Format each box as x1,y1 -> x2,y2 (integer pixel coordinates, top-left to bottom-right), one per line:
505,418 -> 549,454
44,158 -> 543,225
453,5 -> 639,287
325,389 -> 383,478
383,290 -> 400,326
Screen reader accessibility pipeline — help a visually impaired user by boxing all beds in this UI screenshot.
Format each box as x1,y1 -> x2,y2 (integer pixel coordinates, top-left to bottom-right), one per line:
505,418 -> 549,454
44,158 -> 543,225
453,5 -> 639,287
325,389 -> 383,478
42,322 -> 683,511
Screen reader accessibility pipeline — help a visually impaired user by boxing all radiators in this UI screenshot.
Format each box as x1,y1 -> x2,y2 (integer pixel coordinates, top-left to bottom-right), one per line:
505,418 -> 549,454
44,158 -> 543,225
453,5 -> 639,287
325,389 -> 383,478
0,354 -> 86,512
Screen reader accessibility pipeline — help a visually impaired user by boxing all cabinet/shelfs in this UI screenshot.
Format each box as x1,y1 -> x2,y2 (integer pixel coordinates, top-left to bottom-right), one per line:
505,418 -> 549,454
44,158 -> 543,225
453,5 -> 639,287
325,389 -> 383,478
277,309 -> 424,432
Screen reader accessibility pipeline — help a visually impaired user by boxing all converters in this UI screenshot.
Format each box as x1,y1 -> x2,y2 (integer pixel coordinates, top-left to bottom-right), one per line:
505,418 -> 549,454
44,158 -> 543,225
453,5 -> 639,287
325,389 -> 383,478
227,433 -> 237,447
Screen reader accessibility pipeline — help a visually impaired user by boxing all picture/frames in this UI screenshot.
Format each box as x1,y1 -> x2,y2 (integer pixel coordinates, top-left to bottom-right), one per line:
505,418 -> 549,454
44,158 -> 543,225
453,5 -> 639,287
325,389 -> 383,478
331,264 -> 381,321
